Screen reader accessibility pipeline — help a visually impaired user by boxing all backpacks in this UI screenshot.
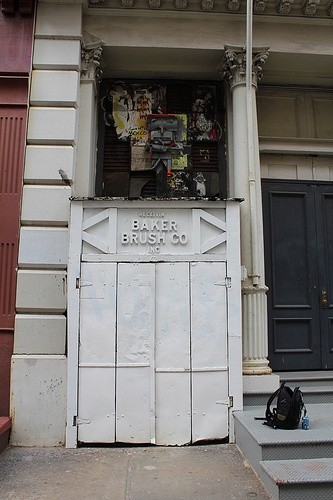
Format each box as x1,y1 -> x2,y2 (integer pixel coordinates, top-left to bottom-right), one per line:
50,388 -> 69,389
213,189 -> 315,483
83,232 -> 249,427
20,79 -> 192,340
254,381 -> 307,430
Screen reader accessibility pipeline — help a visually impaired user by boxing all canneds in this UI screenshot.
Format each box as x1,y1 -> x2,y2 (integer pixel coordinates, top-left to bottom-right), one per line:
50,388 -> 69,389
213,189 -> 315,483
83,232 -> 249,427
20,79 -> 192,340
302,416 -> 309,430
